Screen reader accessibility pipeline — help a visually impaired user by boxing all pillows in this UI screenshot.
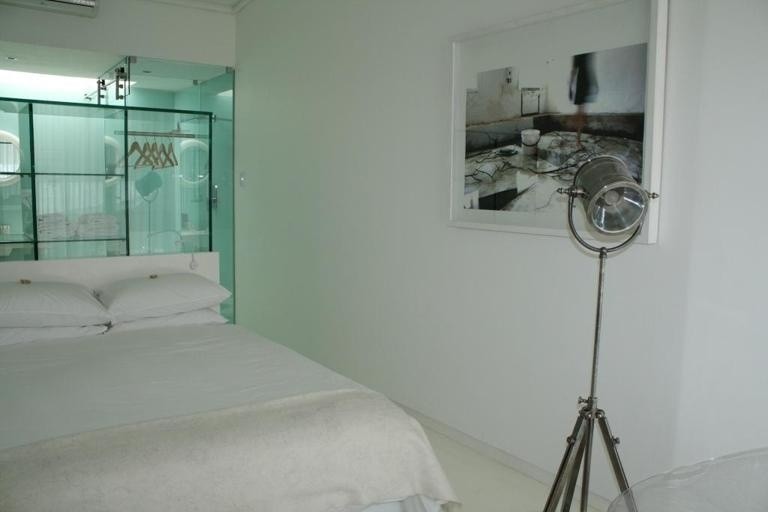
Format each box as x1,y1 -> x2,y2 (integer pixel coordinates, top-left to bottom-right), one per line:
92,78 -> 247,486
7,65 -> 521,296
0,269 -> 236,349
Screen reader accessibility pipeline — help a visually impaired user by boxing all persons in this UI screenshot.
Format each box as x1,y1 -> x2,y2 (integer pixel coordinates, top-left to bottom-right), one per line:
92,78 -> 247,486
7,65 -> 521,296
569,53 -> 598,152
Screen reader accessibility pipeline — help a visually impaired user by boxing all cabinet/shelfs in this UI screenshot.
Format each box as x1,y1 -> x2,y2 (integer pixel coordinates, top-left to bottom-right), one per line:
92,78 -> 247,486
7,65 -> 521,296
0,56 -> 234,328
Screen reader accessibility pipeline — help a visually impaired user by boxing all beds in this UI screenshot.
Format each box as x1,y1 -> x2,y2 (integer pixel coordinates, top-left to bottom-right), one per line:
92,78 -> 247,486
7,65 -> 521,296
0,247 -> 440,512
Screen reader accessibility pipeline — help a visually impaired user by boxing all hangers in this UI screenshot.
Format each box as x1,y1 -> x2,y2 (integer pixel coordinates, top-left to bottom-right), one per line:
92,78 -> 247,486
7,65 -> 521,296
103,132 -> 182,180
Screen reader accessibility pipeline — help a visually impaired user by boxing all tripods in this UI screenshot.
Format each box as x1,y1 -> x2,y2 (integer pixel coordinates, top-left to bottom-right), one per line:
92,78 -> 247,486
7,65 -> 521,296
543,186 -> 659,512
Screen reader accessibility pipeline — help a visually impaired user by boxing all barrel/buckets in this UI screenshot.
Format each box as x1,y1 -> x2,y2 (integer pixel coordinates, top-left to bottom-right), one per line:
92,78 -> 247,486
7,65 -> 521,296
521,128 -> 541,155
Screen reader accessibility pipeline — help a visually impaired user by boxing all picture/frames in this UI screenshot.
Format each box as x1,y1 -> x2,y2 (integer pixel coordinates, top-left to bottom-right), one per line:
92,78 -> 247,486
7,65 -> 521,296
443,0 -> 671,253
